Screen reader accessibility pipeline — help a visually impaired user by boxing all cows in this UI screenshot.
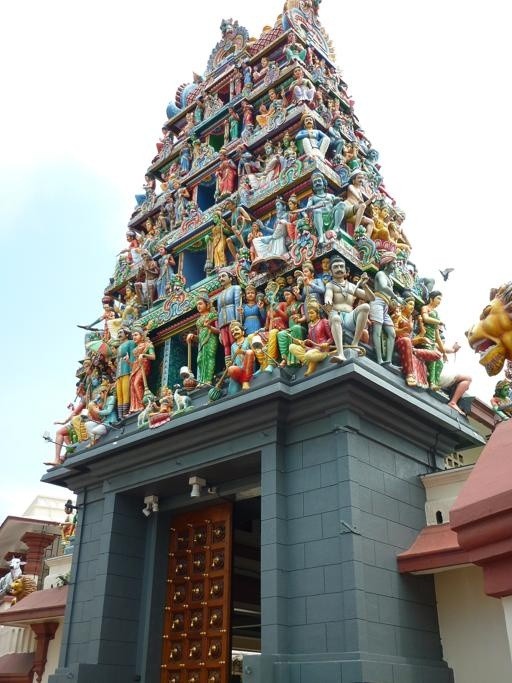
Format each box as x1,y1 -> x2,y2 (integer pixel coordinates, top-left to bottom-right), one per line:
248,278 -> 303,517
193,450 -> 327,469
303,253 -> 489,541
0,554 -> 28,596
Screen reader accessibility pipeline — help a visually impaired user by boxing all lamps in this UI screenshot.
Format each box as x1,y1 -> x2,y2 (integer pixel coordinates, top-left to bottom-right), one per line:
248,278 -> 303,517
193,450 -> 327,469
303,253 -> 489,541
141,496 -> 159,517
38,335 -> 295,449
189,475 -> 206,497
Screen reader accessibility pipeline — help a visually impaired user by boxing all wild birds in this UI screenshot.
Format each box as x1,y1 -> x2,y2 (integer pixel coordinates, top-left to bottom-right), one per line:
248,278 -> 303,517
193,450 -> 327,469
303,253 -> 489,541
438,266 -> 454,281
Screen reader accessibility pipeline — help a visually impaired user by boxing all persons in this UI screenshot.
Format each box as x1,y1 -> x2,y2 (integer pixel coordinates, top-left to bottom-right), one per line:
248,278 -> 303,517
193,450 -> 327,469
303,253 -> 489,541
43,33 -> 471,473
